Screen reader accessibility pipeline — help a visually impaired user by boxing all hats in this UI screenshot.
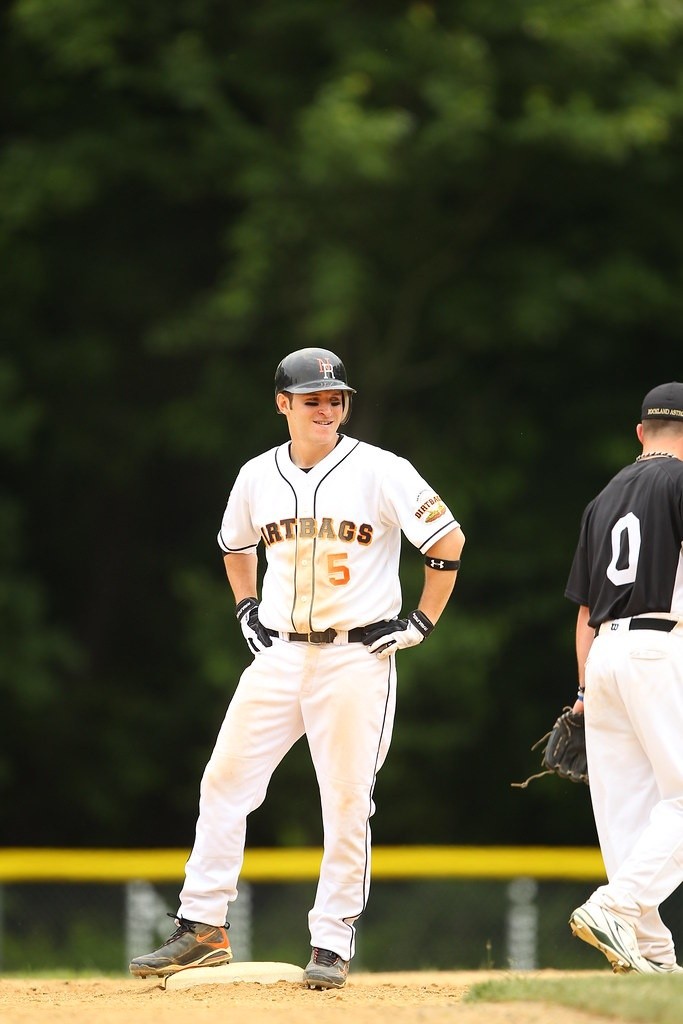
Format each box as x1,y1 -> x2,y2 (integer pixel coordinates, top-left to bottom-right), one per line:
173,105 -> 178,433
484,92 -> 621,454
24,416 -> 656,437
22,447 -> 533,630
641,381 -> 683,420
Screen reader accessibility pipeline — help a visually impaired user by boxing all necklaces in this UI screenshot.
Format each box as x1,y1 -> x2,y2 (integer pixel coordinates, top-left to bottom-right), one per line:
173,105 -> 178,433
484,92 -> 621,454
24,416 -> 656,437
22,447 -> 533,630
636,452 -> 678,461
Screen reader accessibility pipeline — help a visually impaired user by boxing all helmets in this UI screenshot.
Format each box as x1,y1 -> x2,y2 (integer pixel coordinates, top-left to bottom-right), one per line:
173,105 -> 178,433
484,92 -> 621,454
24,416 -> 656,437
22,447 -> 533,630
275,348 -> 357,415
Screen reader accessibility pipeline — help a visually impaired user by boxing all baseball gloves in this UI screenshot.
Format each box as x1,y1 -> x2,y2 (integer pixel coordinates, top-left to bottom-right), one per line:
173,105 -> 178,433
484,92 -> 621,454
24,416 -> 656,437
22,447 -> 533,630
510,705 -> 590,789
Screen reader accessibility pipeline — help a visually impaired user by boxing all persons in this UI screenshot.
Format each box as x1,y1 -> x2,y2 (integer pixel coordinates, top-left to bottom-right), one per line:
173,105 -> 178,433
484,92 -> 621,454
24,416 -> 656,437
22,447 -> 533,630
130,344 -> 466,988
564,383 -> 683,974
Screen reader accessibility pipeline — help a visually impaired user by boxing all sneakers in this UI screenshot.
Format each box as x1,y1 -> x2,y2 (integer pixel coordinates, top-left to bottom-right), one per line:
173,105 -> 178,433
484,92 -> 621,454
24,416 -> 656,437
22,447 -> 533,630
129,913 -> 233,979
303,946 -> 350,991
568,901 -> 683,975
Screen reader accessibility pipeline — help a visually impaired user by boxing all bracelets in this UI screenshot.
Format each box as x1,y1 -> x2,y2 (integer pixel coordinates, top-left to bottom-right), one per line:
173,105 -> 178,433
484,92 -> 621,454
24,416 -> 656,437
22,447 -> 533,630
577,686 -> 585,700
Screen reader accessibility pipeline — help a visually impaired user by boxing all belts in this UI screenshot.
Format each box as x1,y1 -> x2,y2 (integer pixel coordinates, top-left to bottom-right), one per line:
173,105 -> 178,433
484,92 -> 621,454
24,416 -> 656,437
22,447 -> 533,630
266,629 -> 366,645
594,617 -> 677,639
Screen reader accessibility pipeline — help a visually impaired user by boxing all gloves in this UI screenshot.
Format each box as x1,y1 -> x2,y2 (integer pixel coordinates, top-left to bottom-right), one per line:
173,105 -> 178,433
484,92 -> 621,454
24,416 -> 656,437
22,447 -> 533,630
363,609 -> 435,659
235,597 -> 273,655
